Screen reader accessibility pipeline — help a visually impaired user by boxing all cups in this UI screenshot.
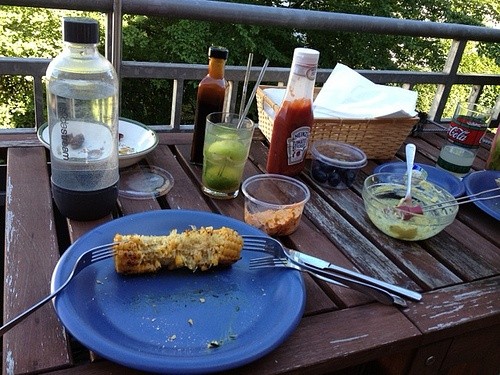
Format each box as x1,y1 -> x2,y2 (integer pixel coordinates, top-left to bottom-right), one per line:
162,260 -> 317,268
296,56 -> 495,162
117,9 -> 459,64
202,112 -> 255,199
435,102 -> 495,177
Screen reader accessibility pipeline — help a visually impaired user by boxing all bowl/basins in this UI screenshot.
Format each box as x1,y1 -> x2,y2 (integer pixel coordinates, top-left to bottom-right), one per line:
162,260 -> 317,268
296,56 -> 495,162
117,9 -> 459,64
312,141 -> 368,190
362,173 -> 458,241
242,174 -> 310,236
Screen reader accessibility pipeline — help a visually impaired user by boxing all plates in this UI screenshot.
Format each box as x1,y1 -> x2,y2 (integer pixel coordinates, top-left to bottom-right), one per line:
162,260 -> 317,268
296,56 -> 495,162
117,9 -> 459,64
51,209 -> 306,373
374,162 -> 465,198
463,171 -> 500,221
37,117 -> 160,168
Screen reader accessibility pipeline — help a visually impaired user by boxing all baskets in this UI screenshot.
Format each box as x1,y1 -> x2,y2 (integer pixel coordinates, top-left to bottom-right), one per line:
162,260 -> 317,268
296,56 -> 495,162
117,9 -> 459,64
256,84 -> 420,161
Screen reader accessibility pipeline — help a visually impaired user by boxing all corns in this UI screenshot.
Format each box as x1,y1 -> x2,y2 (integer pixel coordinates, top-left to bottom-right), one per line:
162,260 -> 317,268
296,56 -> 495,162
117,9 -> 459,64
114,225 -> 244,273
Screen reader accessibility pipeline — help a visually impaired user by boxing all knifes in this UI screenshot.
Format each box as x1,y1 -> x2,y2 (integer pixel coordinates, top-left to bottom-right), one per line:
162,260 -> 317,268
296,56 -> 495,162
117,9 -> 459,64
285,247 -> 422,301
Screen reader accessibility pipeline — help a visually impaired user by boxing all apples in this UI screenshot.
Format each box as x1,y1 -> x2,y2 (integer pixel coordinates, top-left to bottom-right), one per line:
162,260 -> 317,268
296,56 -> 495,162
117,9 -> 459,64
204,134 -> 245,190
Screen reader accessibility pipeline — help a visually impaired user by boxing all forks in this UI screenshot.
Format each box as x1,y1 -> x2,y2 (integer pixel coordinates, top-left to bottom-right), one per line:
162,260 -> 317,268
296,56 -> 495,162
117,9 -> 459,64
249,257 -> 408,307
0,242 -> 120,336
241,235 -> 394,304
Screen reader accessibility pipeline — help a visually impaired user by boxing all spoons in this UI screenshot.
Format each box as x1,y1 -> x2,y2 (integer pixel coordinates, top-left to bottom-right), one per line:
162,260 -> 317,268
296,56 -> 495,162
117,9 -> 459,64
398,143 -> 416,207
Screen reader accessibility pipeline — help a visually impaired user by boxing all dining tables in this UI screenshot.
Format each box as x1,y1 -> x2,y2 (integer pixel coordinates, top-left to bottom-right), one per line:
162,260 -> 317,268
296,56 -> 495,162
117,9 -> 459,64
0,119 -> 500,375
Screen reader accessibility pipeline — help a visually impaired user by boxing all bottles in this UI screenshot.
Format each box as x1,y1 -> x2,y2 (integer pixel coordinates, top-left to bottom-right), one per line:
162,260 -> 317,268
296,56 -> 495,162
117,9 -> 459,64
190,46 -> 232,167
46,16 -> 119,222
267,48 -> 320,177
